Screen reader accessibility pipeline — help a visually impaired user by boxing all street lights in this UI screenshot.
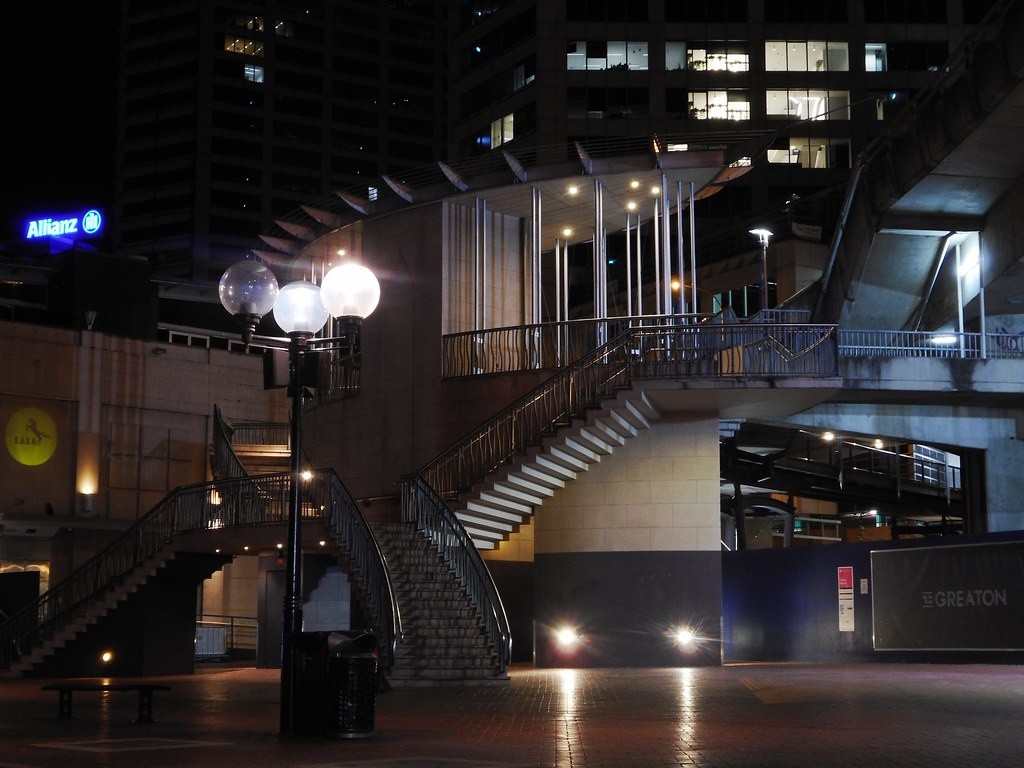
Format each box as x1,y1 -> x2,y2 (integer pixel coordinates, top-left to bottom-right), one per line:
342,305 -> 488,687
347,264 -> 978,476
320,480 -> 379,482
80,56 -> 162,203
218,260 -> 381,739
